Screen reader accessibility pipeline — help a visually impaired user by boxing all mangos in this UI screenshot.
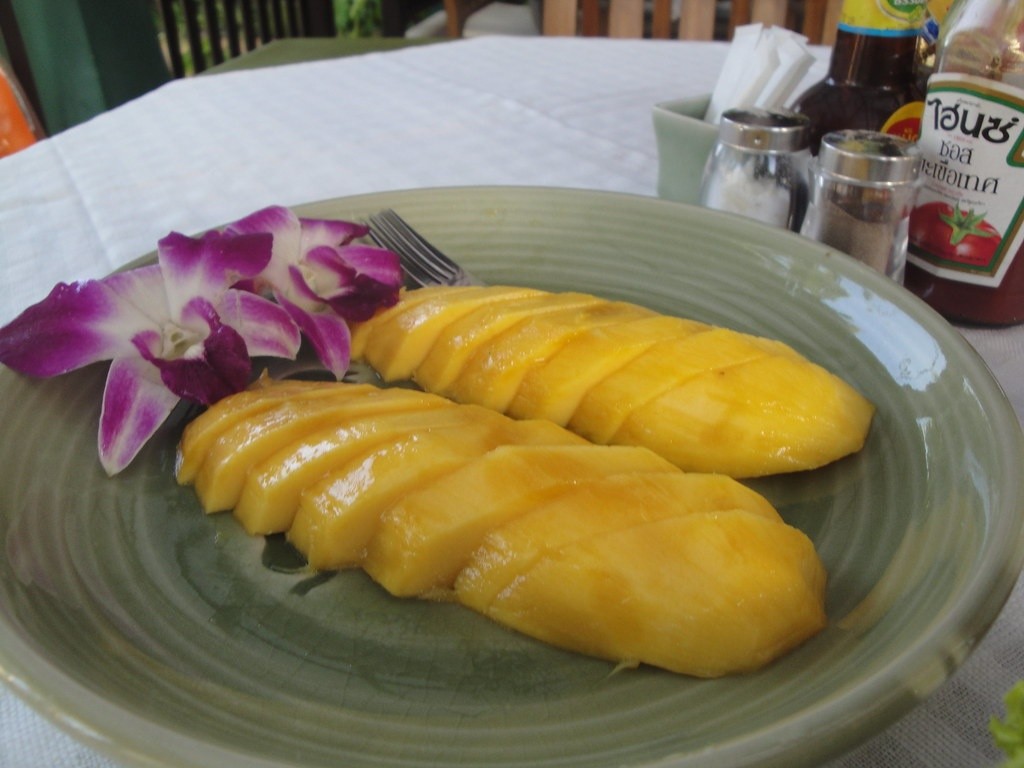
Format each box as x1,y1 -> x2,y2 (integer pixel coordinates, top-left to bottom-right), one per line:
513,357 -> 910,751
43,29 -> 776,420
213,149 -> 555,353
175,377 -> 827,681
340,283 -> 877,478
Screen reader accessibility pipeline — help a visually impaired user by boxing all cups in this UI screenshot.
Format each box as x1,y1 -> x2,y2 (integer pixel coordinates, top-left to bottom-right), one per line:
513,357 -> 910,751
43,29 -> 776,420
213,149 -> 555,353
651,93 -> 720,206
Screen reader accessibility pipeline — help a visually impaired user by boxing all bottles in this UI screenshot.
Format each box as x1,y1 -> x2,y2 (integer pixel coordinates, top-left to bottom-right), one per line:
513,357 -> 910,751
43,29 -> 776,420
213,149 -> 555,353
786,0 -> 927,236
696,108 -> 814,231
906,1 -> 1023,326
799,131 -> 923,287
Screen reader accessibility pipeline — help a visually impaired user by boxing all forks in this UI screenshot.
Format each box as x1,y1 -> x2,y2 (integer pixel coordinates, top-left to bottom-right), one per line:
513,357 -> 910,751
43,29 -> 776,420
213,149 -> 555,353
363,209 -> 470,290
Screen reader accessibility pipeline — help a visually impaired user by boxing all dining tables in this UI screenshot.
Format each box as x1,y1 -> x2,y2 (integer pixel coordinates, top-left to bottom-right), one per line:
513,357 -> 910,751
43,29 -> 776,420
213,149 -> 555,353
0,37 -> 1024,768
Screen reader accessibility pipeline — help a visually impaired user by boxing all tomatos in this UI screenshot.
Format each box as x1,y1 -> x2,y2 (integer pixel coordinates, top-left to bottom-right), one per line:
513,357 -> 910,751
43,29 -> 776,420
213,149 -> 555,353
908,200 -> 1003,269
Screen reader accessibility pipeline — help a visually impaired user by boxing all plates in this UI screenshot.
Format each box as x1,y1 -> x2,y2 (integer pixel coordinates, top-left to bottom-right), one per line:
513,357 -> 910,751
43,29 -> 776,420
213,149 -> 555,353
2,185 -> 1024,768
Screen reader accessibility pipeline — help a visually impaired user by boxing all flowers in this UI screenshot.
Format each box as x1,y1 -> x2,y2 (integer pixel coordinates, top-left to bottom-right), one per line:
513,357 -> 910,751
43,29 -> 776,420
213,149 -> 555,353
1,203 -> 404,477
